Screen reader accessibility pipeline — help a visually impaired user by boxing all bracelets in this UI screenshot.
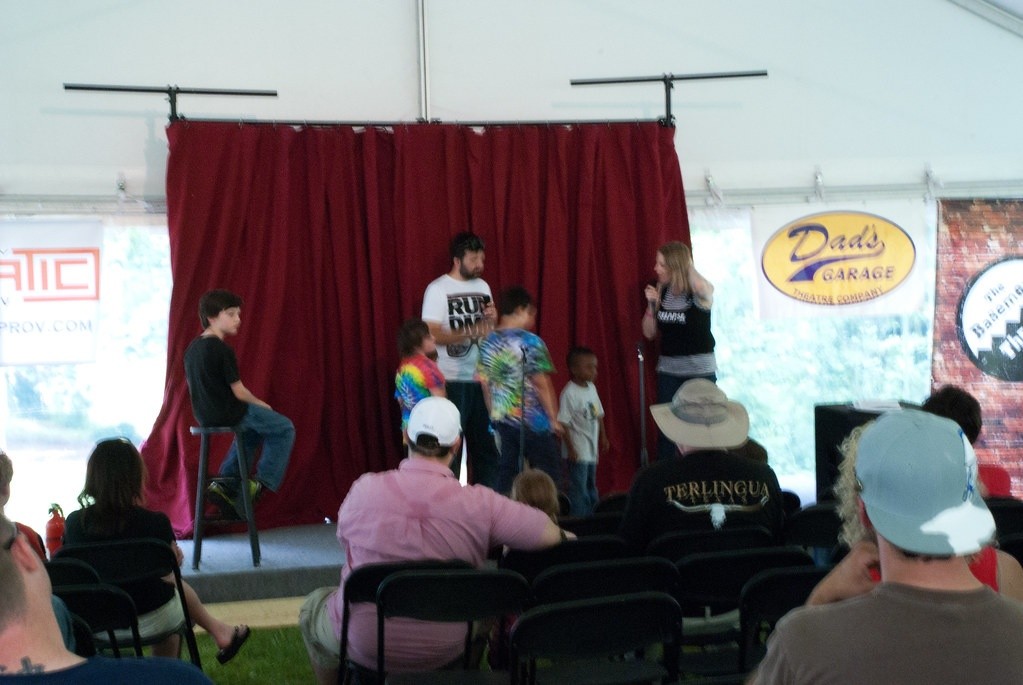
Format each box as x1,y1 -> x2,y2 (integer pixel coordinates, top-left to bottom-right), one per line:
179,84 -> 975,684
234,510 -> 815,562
646,310 -> 653,317
560,529 -> 567,542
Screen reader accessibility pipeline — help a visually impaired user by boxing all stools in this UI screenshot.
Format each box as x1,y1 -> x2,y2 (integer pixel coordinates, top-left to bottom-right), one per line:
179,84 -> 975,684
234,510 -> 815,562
190,424 -> 262,570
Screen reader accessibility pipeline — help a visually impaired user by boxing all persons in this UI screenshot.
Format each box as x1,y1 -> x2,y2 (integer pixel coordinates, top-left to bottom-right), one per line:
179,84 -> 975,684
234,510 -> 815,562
557,348 -> 609,518
628,378 -> 782,558
476,287 -> 564,474
395,323 -> 446,445
422,234 -> 496,485
184,290 -> 295,521
643,243 -> 716,461
754,410 -> 1023,685
300,397 -> 577,685
899,385 -> 982,443
487,470 -> 559,672
0,438 -> 252,685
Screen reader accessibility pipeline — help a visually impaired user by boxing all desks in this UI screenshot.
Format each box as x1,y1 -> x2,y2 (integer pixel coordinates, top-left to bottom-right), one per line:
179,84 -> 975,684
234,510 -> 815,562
816,401 -> 924,501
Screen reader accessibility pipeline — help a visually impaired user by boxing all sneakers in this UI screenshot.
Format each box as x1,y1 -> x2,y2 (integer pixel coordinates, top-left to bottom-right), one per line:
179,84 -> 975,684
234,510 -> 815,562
236,477 -> 262,521
205,480 -> 242,522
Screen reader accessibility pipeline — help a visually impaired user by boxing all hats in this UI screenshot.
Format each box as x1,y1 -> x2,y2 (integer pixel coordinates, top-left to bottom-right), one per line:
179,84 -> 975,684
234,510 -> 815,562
898,386 -> 983,443
648,378 -> 749,450
405,395 -> 464,457
853,409 -> 996,557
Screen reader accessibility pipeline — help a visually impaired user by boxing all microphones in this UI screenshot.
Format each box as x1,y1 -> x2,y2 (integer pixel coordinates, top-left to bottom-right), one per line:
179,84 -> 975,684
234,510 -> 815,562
483,295 -> 490,308
649,278 -> 657,320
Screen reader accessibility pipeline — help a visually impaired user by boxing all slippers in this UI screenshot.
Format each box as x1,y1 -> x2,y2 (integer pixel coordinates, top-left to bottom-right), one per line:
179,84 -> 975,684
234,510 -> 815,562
215,625 -> 250,664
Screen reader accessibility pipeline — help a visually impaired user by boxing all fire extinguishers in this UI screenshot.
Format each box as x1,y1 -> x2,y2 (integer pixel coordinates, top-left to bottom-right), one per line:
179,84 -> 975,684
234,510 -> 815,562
45,504 -> 65,561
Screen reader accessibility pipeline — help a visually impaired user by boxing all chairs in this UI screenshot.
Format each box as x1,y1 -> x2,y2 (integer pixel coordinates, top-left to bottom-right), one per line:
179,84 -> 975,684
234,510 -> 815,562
41,537 -> 204,672
337,491 -> 1023,685
